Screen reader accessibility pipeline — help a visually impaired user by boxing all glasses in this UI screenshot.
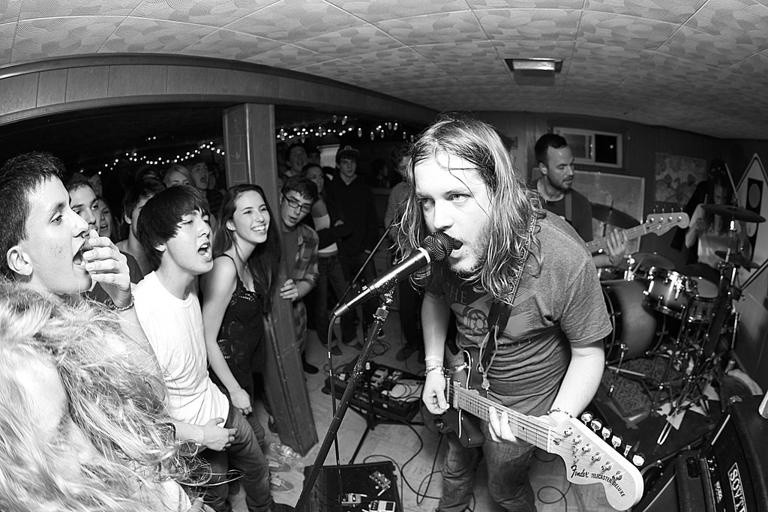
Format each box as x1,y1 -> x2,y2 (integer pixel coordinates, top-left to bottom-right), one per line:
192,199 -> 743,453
282,192 -> 313,214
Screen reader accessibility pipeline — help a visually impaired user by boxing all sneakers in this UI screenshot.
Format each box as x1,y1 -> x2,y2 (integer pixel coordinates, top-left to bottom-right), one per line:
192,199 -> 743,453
248,503 -> 295,512
303,362 -> 318,373
344,342 -> 362,351
269,474 -> 293,493
266,455 -> 290,472
396,346 -> 417,361
322,344 -> 342,355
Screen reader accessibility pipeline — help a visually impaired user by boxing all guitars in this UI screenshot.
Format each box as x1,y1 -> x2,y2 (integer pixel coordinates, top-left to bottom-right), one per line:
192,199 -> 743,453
419,340 -> 644,511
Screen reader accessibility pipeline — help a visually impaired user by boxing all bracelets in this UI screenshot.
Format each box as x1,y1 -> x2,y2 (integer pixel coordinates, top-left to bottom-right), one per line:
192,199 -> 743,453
424,364 -> 445,377
546,408 -> 572,419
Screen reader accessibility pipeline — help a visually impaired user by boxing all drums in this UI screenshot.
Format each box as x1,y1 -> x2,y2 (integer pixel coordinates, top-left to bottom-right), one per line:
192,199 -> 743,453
684,274 -> 719,323
641,266 -> 697,319
599,279 -> 667,364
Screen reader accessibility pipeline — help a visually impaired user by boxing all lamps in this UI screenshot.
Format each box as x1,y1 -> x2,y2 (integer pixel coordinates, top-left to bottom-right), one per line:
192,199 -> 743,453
504,57 -> 562,74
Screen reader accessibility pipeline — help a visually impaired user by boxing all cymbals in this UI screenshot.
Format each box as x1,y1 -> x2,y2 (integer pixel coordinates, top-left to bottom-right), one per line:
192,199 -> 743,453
631,252 -> 674,266
715,250 -> 762,269
590,199 -> 640,229
701,203 -> 765,223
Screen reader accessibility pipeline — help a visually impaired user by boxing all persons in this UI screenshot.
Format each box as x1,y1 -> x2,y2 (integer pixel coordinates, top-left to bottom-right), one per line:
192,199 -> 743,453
527,133 -> 628,269
685,176 -> 733,272
387,116 -> 612,512
0,145 -> 424,512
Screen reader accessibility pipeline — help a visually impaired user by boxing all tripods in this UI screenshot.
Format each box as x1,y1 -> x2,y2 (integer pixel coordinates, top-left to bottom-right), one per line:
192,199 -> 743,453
606,264 -> 738,450
344,281 -> 423,466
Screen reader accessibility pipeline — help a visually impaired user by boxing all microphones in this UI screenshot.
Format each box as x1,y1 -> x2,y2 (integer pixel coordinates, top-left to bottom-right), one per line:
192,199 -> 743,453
332,233 -> 453,317
